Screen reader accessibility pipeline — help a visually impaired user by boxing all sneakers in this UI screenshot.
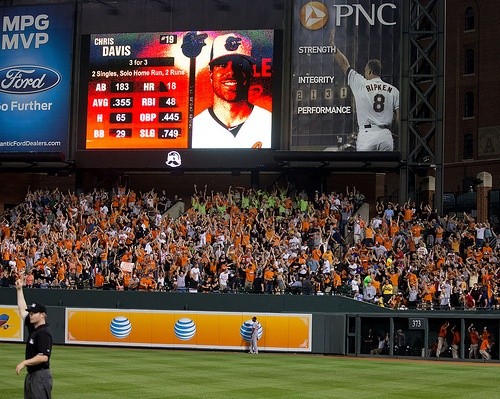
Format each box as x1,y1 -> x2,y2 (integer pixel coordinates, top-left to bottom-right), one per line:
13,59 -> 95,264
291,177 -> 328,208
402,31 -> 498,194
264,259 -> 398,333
254,352 -> 258,354
248,351 -> 253,354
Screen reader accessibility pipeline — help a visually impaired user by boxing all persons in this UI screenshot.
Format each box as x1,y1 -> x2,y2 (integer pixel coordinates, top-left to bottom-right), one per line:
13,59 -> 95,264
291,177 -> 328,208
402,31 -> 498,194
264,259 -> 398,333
189,34 -> 271,149
14,274 -> 54,399
329,28 -> 400,152
0,182 -> 500,361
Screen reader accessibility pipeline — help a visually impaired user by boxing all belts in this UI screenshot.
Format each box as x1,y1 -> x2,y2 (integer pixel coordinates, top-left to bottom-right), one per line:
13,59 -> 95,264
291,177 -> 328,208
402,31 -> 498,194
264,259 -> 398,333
27,365 -> 49,373
364,125 -> 387,129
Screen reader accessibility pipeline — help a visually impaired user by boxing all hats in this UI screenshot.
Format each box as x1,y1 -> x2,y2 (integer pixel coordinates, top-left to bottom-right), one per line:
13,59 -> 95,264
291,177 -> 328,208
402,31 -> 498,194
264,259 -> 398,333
208,32 -> 252,66
25,300 -> 47,313
